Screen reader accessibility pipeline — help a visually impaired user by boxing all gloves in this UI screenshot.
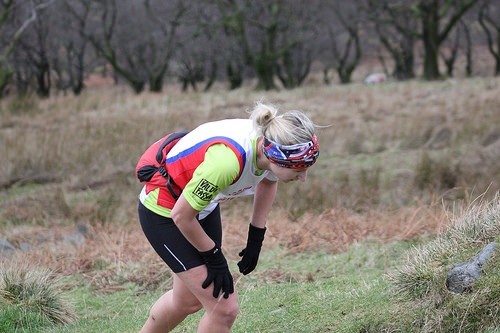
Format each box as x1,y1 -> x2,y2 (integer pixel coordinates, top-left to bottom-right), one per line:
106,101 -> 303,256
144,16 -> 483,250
236,223 -> 267,276
198,241 -> 234,299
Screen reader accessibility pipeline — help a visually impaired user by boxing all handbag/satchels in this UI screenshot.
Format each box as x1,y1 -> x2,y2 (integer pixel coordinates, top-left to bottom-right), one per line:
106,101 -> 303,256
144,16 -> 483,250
133,127 -> 195,203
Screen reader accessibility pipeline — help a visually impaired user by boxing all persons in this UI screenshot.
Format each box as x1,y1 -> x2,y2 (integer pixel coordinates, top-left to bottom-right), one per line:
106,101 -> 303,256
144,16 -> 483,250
138,104 -> 320,333
